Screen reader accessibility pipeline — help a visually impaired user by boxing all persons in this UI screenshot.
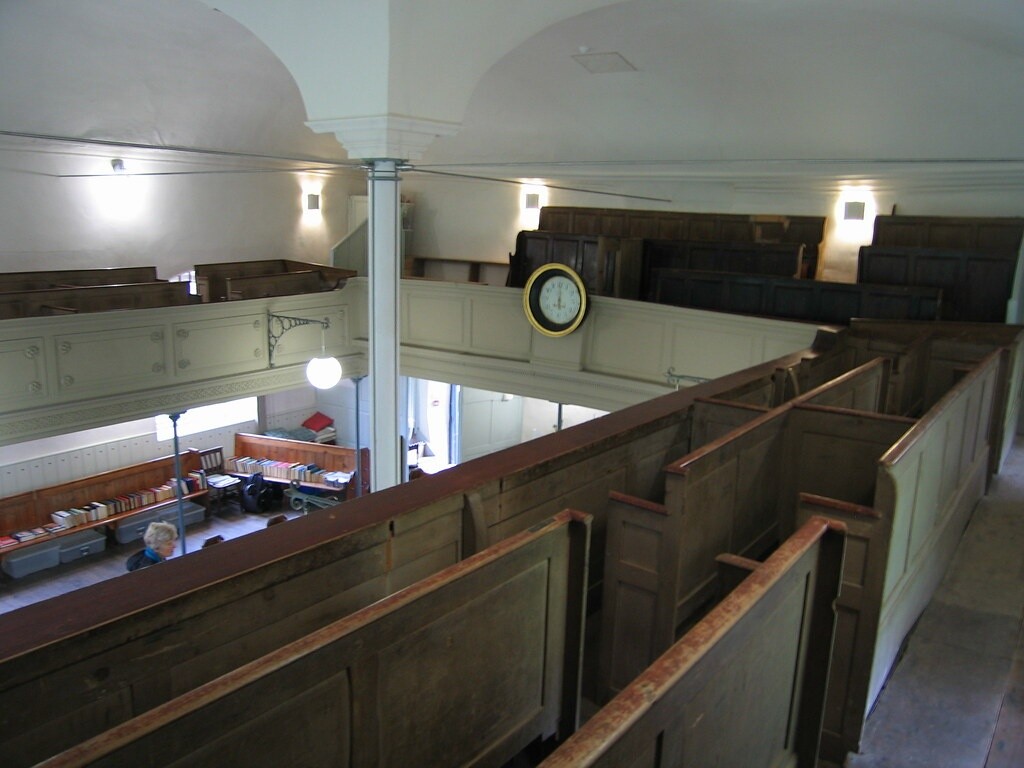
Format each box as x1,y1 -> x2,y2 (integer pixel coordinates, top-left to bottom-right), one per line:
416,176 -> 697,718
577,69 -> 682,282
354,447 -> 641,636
125,520 -> 178,572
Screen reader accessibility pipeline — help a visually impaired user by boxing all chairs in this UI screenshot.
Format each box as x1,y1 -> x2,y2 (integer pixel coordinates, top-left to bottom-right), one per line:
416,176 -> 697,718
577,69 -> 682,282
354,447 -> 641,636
199,445 -> 244,517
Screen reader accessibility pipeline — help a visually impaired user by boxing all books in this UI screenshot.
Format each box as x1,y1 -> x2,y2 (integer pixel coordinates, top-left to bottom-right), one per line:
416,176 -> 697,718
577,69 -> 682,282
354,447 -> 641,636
224,454 -> 352,489
1,471 -> 208,551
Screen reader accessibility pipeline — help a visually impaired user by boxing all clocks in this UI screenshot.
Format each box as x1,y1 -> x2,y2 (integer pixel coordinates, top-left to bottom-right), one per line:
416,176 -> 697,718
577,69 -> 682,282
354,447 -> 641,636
523,262 -> 587,338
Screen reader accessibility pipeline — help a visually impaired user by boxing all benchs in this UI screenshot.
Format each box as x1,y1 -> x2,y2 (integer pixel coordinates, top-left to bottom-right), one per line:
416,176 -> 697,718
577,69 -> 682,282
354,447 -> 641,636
224,433 -> 370,501
218,269 -> 334,302
193,260 -> 287,303
0,448 -> 212,577
0,266 -> 170,291
0,280 -> 203,320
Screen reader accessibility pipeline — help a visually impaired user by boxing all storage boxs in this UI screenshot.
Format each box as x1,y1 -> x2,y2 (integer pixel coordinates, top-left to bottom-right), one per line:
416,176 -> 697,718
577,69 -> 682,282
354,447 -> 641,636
159,502 -> 206,529
115,513 -> 160,545
0,540 -> 60,580
59,531 -> 107,564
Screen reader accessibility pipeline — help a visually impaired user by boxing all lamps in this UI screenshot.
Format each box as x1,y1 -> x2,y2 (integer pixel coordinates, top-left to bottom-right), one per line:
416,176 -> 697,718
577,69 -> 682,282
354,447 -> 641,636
308,193 -> 319,210
266,308 -> 343,389
526,193 -> 540,209
843,200 -> 865,221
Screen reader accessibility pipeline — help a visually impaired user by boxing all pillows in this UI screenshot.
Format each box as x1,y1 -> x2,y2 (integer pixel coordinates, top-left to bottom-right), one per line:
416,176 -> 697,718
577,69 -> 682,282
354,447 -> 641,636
301,411 -> 334,432
288,427 -> 317,442
264,426 -> 291,439
